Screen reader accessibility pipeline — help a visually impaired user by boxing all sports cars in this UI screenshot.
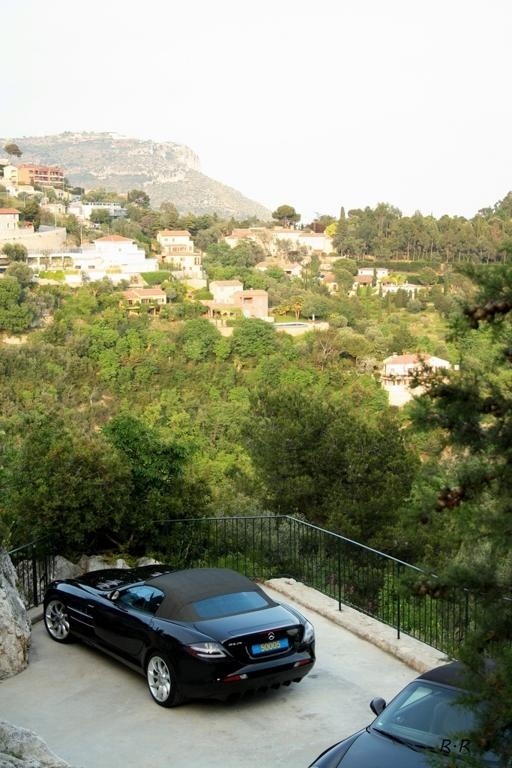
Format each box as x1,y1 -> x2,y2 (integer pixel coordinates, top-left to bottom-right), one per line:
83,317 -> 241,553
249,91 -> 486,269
42,563 -> 317,708
306,658 -> 511,767
